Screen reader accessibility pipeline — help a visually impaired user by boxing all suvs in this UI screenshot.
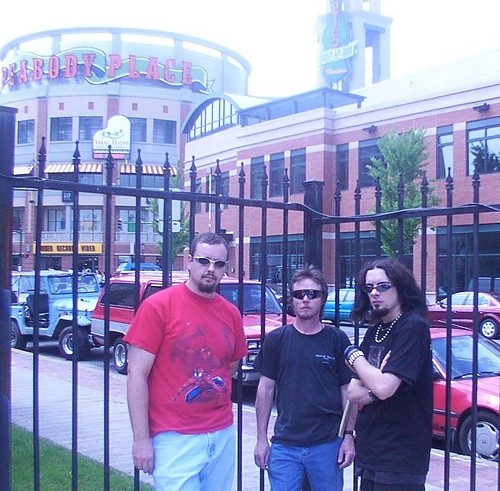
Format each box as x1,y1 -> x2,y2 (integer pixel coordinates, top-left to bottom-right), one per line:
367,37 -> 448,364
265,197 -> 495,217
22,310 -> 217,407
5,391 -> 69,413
10,260 -> 164,362
90,270 -> 296,403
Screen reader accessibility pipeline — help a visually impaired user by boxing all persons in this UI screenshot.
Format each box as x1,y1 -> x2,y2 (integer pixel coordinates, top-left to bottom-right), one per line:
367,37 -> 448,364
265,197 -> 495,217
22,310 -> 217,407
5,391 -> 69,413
252,263 -> 358,491
102,272 -> 105,281
47,265 -> 54,270
121,231 -> 248,491
96,271 -> 103,283
340,259 -> 433,491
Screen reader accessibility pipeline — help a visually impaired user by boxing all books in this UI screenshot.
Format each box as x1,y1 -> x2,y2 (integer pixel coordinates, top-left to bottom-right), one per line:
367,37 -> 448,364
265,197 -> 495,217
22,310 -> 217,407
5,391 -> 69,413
338,400 -> 352,437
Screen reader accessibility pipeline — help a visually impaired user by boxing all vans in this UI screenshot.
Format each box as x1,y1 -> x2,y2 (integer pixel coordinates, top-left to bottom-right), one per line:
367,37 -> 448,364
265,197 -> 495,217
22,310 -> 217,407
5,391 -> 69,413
467,276 -> 500,294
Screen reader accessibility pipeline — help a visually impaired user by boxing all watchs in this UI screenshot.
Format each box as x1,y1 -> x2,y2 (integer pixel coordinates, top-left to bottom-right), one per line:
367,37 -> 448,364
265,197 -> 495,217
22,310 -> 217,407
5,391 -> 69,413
344,429 -> 356,438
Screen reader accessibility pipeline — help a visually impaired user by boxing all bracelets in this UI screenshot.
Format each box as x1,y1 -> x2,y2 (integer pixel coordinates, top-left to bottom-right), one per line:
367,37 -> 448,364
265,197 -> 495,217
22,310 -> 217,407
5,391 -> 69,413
348,350 -> 365,366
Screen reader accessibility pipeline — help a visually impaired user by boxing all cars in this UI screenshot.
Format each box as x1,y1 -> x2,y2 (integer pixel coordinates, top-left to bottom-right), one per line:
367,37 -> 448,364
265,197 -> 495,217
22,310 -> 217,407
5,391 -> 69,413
427,290 -> 500,341
321,287 -> 366,328
232,282 -> 294,316
348,319 -> 500,462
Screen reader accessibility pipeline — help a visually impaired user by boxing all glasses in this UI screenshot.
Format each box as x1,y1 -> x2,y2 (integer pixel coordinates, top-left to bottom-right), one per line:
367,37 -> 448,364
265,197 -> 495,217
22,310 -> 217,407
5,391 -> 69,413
291,289 -> 323,299
192,256 -> 228,268
361,283 -> 397,294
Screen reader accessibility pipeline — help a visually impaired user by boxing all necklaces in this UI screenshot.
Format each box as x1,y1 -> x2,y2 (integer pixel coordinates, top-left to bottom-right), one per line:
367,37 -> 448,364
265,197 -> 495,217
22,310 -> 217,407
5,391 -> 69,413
375,312 -> 403,343
320,323 -> 324,328
